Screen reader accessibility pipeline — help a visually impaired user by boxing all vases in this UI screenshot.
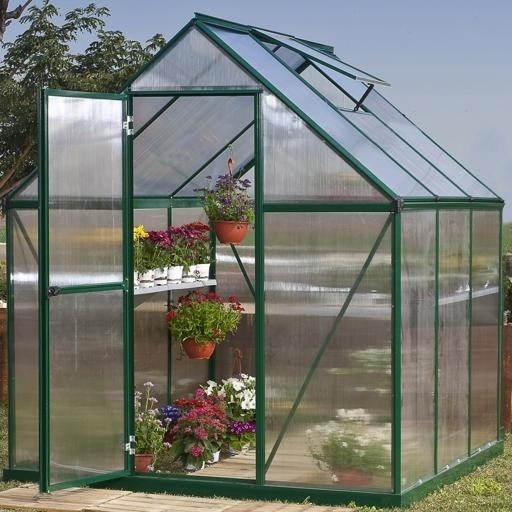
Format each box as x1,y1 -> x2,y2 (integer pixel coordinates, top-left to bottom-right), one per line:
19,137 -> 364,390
333,467 -> 373,486
132,263 -> 210,289
182,338 -> 217,361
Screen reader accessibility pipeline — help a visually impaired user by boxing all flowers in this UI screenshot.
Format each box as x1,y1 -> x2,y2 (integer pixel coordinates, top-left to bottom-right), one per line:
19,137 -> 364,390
131,372 -> 255,472
305,408 -> 391,478
165,291 -> 245,342
132,173 -> 255,270
